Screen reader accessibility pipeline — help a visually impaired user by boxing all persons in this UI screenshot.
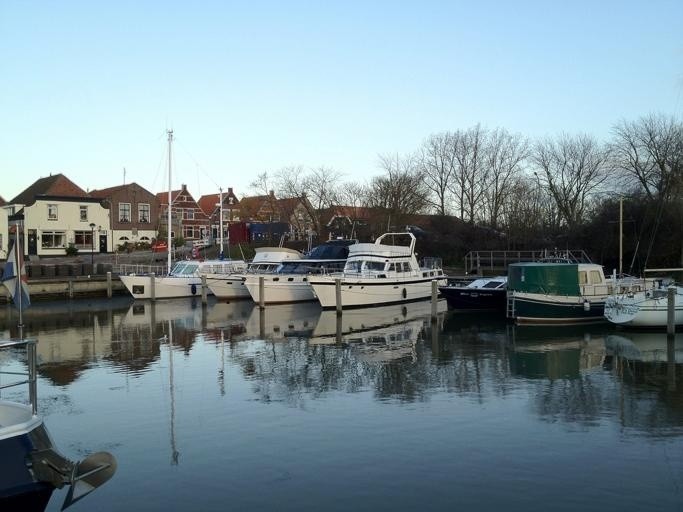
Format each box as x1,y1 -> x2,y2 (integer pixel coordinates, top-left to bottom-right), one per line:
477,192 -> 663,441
217,251 -> 226,261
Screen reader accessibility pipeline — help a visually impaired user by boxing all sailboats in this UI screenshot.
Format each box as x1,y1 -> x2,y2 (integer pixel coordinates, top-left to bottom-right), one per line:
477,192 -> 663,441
604,328 -> 683,434
117,127 -> 249,300
602,191 -> 683,328
118,298 -> 207,468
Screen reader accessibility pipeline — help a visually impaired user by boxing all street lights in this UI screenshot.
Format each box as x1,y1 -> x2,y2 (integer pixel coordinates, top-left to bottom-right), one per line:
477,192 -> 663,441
88,222 -> 96,264
90,311 -> 100,370
216,327 -> 226,396
217,186 -> 224,260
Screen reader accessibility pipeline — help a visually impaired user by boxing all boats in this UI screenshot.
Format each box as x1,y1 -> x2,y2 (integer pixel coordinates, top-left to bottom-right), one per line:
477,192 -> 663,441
194,258 -> 253,300
246,299 -> 323,363
203,298 -> 260,349
0,338 -> 117,512
505,257 -> 677,327
506,327 -> 606,382
436,275 -> 508,310
308,231 -> 449,309
242,236 -> 360,305
307,293 -> 449,365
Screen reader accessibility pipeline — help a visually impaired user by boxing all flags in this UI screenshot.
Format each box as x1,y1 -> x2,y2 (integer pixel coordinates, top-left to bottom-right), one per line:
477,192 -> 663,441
0,227 -> 31,315
